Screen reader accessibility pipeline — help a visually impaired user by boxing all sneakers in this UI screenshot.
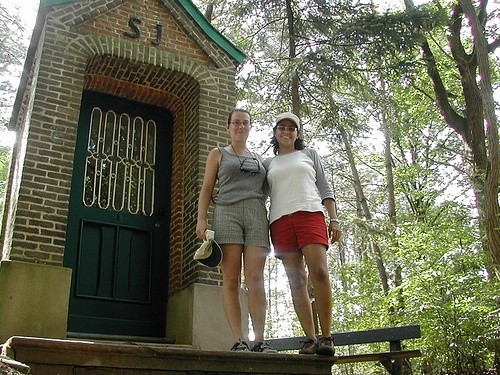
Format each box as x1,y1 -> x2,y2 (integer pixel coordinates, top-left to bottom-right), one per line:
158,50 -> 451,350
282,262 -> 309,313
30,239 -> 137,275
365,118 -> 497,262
299,339 -> 319,354
316,336 -> 335,355
251,342 -> 277,353
230,340 -> 250,352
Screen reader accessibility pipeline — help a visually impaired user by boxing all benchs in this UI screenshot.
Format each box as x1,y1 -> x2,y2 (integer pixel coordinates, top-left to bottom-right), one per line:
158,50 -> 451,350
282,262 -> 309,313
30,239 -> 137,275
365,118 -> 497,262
249,324 -> 422,374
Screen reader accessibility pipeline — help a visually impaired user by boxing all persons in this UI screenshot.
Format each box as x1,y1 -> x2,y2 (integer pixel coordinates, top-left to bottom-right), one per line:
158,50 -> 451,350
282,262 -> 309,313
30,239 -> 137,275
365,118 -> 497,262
196,109 -> 278,352
262,113 -> 341,355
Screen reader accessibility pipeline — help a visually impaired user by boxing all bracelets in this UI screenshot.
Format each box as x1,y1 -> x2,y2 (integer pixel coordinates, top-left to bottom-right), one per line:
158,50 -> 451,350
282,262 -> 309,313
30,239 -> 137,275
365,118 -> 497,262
329,219 -> 340,223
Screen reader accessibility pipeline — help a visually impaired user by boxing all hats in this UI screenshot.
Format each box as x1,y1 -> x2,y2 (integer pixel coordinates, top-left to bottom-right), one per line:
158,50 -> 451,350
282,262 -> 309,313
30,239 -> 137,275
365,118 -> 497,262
276,113 -> 300,131
194,239 -> 224,269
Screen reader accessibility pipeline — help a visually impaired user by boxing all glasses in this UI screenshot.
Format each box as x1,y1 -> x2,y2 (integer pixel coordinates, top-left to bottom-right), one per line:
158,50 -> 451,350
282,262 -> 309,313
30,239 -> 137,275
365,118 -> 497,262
230,119 -> 251,128
241,158 -> 260,177
274,126 -> 298,133
